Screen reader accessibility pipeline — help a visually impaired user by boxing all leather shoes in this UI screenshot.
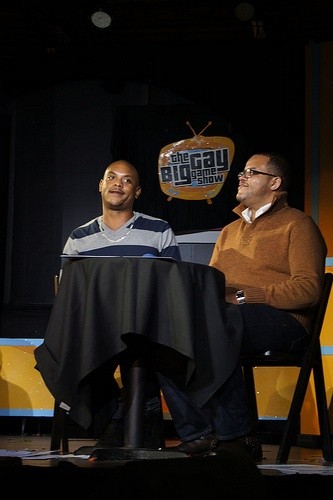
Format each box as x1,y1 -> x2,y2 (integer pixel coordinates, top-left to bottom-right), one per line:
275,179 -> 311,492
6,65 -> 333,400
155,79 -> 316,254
205,438 -> 261,462
159,436 -> 210,456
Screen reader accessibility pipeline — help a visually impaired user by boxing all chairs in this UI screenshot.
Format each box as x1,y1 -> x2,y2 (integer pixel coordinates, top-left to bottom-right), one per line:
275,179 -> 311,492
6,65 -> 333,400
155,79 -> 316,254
244,273 -> 333,464
50,271 -> 99,454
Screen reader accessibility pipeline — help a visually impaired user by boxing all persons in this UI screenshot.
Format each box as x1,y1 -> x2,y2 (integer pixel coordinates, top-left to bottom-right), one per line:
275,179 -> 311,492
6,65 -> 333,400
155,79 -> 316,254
57,159 -> 182,454
160,151 -> 328,462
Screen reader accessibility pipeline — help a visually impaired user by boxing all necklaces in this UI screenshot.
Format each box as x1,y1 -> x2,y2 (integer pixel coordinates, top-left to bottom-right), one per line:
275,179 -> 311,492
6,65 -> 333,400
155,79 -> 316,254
99,211 -> 136,242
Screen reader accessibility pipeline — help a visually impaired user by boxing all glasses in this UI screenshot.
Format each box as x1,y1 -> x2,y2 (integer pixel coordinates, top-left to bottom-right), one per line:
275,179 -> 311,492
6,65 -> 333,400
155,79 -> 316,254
238,169 -> 278,179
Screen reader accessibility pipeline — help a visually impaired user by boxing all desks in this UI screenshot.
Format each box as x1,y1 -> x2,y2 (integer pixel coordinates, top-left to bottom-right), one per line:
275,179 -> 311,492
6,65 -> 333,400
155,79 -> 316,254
34,259 -> 241,460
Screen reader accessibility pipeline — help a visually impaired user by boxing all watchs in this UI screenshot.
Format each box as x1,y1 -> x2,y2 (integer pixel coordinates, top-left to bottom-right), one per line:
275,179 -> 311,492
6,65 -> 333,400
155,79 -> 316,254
236,290 -> 245,304
